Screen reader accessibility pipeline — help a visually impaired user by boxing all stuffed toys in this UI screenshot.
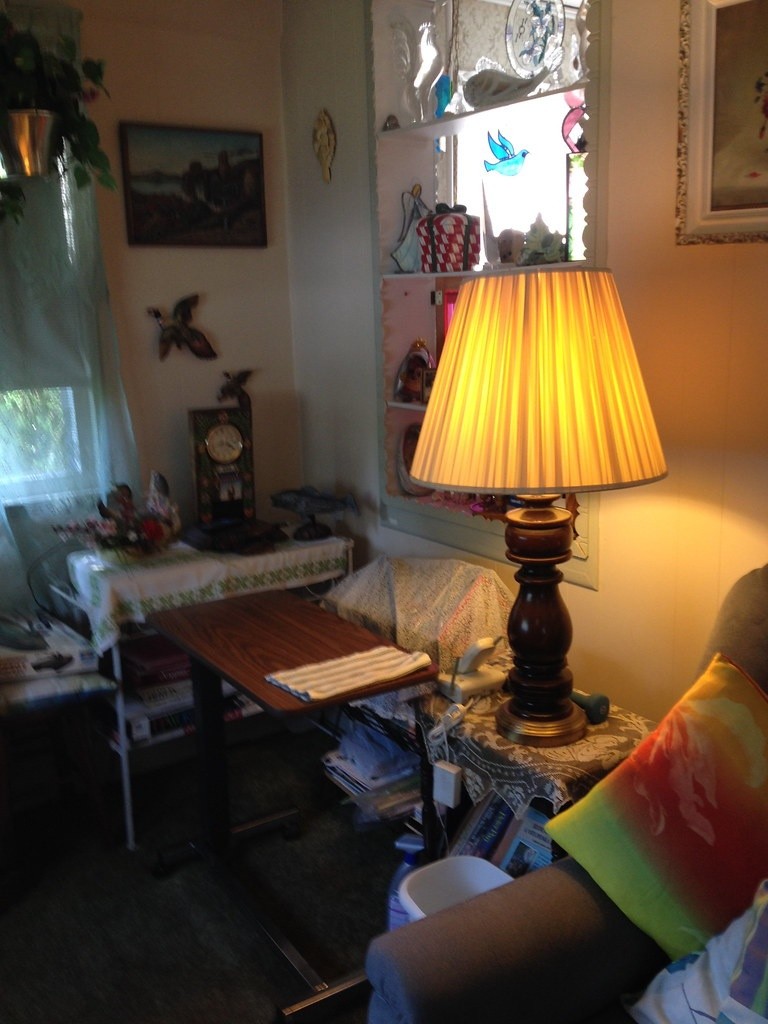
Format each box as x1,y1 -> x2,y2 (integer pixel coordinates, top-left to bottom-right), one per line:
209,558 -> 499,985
400,356 -> 427,402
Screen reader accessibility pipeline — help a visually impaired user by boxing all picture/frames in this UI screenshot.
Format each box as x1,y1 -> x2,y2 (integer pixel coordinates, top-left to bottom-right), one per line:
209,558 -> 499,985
117,120 -> 271,249
674,0 -> 768,245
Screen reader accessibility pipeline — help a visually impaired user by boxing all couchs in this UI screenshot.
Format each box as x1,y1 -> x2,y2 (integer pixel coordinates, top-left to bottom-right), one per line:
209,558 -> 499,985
364,563 -> 768,1024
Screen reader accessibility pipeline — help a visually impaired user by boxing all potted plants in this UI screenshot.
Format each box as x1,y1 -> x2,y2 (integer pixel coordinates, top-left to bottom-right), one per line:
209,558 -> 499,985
0,12 -> 116,228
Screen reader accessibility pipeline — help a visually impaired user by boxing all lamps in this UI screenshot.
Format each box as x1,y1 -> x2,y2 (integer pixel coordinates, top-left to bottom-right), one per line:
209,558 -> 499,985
411,265 -> 670,747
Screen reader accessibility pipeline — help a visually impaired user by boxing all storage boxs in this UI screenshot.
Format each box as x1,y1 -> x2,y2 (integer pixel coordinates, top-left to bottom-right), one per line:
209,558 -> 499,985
0,608 -> 101,684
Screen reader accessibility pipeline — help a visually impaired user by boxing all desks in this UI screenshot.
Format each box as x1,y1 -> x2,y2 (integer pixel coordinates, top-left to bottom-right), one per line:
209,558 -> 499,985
145,588 -> 441,1024
66,515 -> 360,853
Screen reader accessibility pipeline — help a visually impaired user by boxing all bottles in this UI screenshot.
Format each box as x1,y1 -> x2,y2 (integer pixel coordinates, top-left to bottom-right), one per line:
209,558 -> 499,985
388,854 -> 416,932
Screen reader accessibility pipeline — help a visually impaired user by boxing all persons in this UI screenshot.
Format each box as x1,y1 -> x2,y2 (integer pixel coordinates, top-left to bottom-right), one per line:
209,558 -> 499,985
182,148 -> 242,230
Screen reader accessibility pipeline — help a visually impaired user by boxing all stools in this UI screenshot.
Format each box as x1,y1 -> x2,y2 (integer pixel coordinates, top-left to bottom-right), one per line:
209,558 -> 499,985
405,685 -> 661,882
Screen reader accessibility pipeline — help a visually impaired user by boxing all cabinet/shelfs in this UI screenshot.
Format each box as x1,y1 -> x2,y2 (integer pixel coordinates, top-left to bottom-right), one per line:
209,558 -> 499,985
365,0 -> 615,594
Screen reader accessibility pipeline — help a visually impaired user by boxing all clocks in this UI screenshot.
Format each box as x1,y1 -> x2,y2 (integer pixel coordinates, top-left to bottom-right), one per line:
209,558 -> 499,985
188,405 -> 258,527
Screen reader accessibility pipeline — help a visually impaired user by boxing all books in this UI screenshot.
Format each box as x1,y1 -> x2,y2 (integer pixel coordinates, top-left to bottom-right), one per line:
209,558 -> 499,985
324,748 -> 421,810
410,800 -> 446,825
448,785 -> 557,881
124,646 -> 194,709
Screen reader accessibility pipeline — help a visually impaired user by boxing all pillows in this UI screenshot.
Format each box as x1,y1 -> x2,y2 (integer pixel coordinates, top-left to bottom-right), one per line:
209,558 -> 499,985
541,652 -> 768,962
621,878 -> 768,1024
